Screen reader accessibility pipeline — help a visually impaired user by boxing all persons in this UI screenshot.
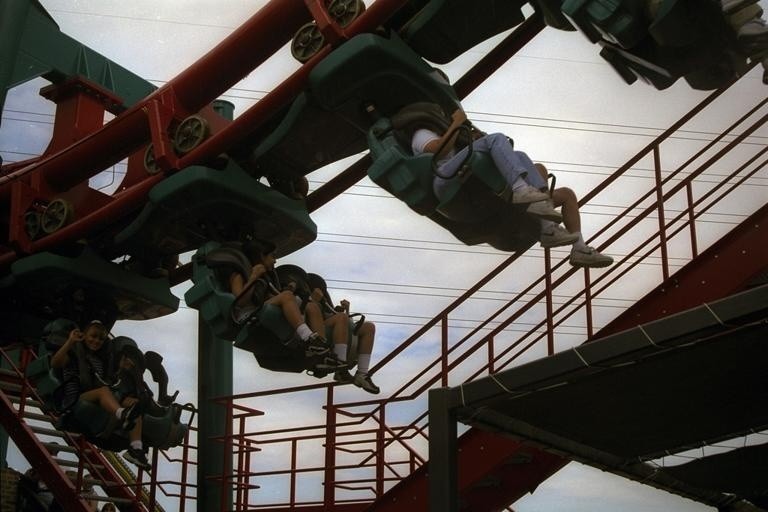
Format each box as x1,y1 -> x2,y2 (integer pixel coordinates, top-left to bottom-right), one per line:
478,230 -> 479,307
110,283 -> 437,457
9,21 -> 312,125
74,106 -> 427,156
411,108 -> 563,224
535,163 -> 613,268
229,239 -> 350,369
310,288 -> 380,394
49,320 -> 151,471
24,442 -> 116,512
645,0 -> 768,84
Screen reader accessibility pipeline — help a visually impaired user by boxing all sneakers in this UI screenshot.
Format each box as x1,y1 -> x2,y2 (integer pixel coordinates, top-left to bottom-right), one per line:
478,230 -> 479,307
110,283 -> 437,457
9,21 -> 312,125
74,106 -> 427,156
354,370 -> 380,394
569,246 -> 613,268
737,20 -> 768,44
302,331 -> 352,385
118,402 -> 144,431
512,184 -> 551,207
526,200 -> 564,224
540,224 -> 579,248
122,446 -> 152,471
